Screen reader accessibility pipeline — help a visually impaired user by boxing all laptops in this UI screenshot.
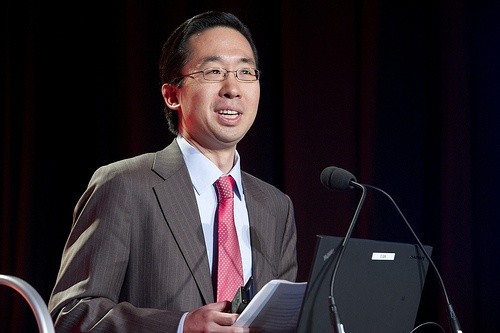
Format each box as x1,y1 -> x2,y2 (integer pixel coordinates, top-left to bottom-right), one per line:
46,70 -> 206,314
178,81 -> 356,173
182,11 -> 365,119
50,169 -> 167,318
295,235 -> 434,333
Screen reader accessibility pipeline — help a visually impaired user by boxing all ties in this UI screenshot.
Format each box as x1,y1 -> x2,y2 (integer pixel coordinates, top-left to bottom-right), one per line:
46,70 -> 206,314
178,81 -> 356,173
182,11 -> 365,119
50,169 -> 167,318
212,176 -> 243,302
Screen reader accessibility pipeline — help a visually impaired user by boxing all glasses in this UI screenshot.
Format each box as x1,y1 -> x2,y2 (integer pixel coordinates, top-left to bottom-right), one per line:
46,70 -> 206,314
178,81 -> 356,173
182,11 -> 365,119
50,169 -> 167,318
170,66 -> 259,84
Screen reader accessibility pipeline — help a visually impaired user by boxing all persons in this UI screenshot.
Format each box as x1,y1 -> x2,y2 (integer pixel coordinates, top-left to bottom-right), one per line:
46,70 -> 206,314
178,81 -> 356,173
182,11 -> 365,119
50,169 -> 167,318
46,12 -> 297,333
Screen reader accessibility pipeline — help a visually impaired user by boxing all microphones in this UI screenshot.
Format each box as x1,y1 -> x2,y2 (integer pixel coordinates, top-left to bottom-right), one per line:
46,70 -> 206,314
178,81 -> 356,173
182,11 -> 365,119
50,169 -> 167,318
320,165 -> 463,333
